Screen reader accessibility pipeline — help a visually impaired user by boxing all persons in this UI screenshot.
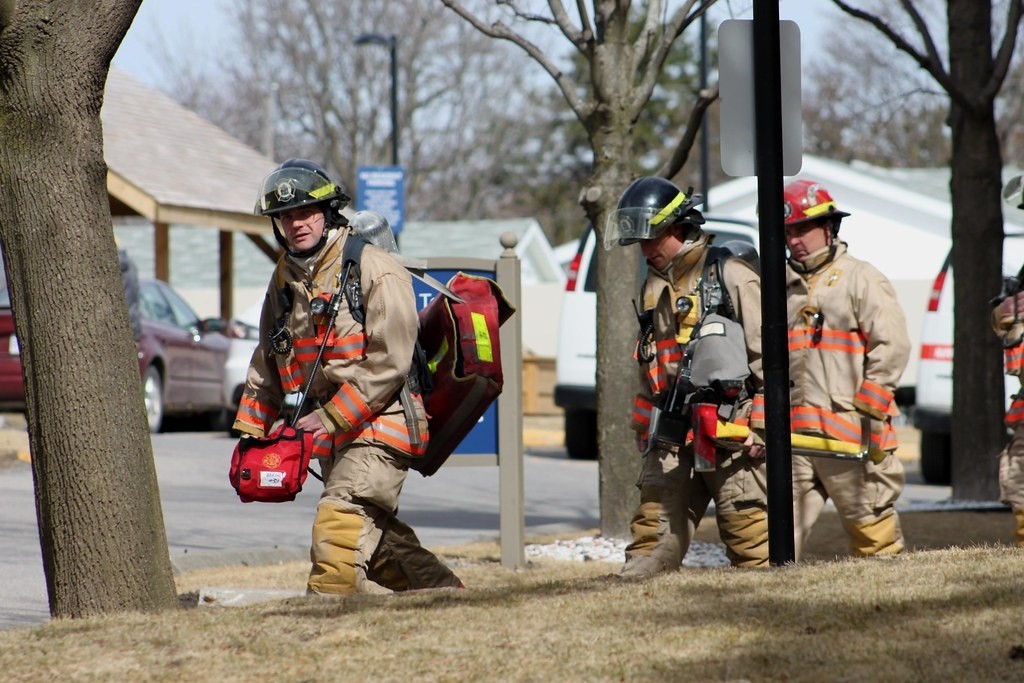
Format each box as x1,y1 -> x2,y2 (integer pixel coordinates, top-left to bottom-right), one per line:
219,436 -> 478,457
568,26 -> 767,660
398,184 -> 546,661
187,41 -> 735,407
783,180 -> 912,558
989,266 -> 1024,548
232,158 -> 465,597
603,176 -> 770,577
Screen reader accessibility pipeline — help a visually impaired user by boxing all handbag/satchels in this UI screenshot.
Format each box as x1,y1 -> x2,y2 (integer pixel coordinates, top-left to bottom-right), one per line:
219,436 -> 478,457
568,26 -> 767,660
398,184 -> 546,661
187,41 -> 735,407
229,424 -> 312,503
411,272 -> 517,477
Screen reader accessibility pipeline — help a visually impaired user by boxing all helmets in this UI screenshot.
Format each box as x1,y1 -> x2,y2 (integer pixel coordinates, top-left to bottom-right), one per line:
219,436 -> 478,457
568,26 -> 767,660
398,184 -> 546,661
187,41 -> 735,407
605,175 -> 694,250
255,155 -> 343,215
784,179 -> 851,223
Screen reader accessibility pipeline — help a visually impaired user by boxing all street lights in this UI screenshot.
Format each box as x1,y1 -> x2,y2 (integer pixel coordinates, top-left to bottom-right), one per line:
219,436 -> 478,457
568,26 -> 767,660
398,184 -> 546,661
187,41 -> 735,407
352,33 -> 400,248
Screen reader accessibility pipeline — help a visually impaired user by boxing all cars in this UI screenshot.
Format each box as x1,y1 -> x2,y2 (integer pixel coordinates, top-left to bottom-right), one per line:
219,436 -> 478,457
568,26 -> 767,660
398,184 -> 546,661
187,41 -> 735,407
0,276 -> 232,433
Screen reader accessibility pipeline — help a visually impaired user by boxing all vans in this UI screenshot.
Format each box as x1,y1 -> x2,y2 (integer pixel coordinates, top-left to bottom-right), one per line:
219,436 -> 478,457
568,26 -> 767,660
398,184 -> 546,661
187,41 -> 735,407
555,214 -> 762,461
914,225 -> 1024,487
